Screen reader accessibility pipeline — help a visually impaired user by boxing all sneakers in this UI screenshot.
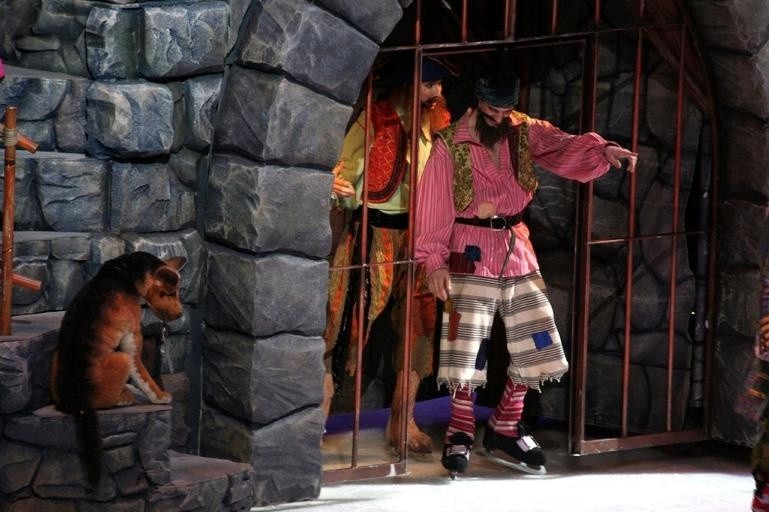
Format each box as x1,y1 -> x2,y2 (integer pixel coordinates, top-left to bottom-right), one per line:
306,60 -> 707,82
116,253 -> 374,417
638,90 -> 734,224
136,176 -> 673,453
441,431 -> 475,473
482,421 -> 546,466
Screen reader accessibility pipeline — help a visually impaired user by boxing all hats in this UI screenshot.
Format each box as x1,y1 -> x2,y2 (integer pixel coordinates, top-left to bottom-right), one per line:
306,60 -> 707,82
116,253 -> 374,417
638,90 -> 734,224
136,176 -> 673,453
402,53 -> 462,83
474,61 -> 521,108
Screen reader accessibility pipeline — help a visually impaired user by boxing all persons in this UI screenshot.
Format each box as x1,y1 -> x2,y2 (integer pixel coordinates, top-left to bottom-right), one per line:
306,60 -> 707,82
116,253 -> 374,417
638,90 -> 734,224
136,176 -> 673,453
411,65 -> 642,474
322,65 -> 453,455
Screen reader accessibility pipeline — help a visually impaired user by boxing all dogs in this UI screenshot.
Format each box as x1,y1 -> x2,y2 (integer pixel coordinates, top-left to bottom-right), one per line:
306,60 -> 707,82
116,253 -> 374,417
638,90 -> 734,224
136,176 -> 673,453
51,251 -> 188,506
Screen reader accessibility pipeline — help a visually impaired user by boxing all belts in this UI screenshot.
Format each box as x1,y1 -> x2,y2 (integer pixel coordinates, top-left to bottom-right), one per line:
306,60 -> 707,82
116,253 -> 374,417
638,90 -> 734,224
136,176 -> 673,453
456,211 -> 523,233
